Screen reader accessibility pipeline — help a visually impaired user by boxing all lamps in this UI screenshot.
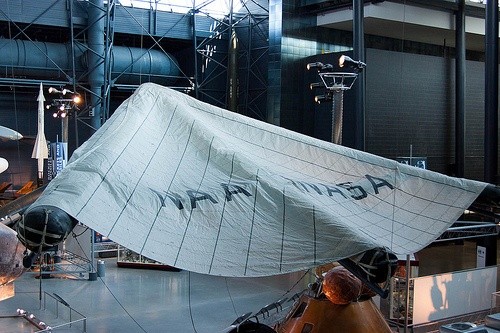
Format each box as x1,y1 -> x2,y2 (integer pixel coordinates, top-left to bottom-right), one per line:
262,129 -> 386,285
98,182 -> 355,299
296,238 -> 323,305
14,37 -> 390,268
307,62 -> 335,73
338,55 -> 367,71
309,81 -> 328,89
46,101 -> 56,109
47,87 -> 62,95
61,88 -> 76,96
51,111 -> 57,119
60,112 -> 69,119
315,93 -> 333,104
59,104 -> 67,111
